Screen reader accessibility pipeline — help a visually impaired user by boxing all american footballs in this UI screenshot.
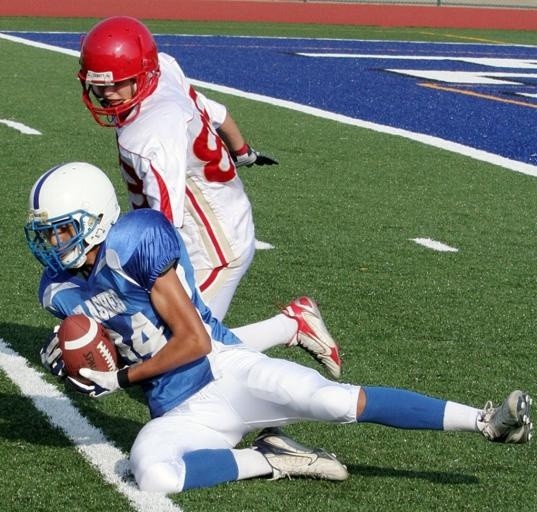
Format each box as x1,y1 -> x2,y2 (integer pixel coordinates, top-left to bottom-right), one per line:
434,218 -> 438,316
58,314 -> 118,388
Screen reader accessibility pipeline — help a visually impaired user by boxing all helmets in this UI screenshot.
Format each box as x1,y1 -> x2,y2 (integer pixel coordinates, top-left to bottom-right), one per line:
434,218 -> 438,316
24,160 -> 121,279
77,15 -> 160,128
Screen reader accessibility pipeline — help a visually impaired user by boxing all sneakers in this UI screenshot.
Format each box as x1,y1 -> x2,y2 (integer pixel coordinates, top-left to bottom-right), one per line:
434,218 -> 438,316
475,390 -> 532,444
250,427 -> 350,482
278,296 -> 341,378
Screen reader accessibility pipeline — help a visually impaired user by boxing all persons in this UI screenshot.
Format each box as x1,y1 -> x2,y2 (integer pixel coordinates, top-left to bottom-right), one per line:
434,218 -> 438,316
74,13 -> 342,382
23,160 -> 535,495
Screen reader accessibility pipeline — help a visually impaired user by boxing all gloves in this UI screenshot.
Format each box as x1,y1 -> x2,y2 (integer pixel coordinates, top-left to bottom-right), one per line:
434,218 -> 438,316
231,144 -> 278,169
67,367 -> 130,399
39,325 -> 65,380
